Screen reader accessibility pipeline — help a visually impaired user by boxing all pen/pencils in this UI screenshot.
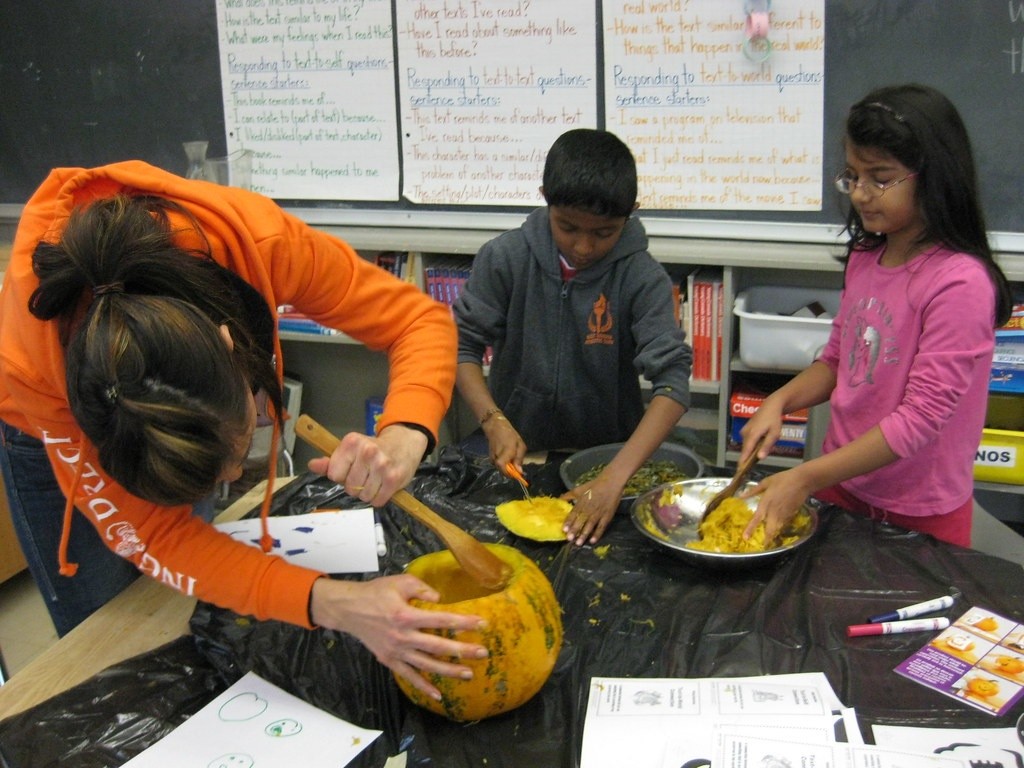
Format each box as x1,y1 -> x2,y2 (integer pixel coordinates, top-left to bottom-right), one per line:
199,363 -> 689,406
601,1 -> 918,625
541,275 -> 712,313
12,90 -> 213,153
848,594 -> 953,637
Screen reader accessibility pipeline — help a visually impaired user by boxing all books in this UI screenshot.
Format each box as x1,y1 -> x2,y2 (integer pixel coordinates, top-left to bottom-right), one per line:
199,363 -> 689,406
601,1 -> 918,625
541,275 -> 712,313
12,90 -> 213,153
273,299 -> 340,337
426,252 -> 474,304
375,248 -> 410,280
665,270 -> 722,382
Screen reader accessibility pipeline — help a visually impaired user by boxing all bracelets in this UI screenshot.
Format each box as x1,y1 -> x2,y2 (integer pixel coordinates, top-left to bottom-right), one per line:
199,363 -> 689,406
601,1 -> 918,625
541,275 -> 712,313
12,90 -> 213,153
480,409 -> 502,425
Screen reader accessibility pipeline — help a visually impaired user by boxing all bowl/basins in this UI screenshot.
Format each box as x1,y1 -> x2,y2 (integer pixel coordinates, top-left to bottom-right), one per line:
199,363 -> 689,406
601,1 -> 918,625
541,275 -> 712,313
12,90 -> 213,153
559,441 -> 704,516
630,477 -> 822,564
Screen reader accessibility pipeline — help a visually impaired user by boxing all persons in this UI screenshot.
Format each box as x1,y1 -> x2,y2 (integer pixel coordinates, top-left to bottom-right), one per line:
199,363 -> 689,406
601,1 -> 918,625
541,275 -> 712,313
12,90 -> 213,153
0,160 -> 491,701
738,84 -> 1014,549
451,128 -> 694,546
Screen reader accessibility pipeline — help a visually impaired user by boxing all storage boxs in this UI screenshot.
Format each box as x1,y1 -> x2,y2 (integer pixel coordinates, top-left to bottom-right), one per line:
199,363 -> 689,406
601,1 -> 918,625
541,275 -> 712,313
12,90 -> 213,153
735,283 -> 843,368
727,384 -> 811,457
970,303 -> 1024,487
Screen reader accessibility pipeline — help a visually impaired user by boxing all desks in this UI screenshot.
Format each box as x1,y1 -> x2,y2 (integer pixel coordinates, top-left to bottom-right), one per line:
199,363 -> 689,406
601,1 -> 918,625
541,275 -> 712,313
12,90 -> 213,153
0,449 -> 1024,766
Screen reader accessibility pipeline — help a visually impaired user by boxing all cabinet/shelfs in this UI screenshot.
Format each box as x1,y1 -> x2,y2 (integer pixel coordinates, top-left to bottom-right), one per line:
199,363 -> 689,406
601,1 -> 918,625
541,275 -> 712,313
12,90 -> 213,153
274,222 -> 1024,501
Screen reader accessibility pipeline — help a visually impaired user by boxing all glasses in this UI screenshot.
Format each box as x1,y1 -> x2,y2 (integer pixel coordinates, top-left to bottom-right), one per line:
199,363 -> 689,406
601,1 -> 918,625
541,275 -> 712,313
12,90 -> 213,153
834,168 -> 922,198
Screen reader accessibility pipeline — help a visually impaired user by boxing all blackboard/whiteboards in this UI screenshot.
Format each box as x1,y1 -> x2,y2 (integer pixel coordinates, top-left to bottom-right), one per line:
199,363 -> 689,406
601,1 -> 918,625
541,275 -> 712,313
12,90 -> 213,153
0,1 -> 1024,254
